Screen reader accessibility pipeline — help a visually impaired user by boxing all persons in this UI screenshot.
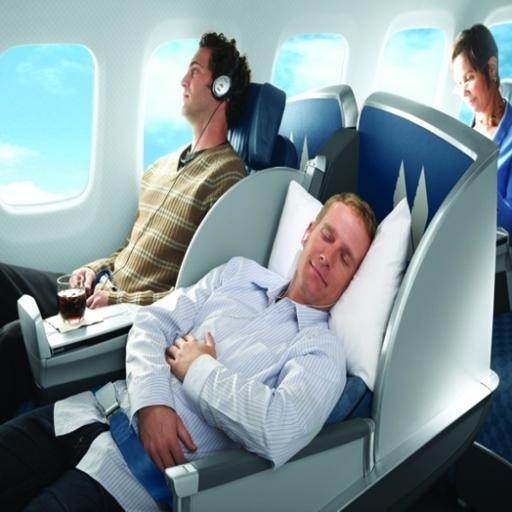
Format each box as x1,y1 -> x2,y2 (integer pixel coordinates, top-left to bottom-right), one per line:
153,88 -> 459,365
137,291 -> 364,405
450,23 -> 512,318
0,192 -> 378,512
0,32 -> 252,421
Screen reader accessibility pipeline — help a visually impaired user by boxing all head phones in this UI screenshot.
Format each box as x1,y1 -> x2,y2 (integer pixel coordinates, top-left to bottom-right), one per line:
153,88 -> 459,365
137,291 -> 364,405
211,56 -> 241,101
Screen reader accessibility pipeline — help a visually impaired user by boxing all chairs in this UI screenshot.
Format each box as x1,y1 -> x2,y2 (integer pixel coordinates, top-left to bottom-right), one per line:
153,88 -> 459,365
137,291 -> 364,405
16,79 -> 512,511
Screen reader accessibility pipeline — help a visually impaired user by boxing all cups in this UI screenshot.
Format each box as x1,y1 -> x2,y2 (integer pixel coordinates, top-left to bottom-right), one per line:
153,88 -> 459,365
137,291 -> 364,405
56,274 -> 86,326
